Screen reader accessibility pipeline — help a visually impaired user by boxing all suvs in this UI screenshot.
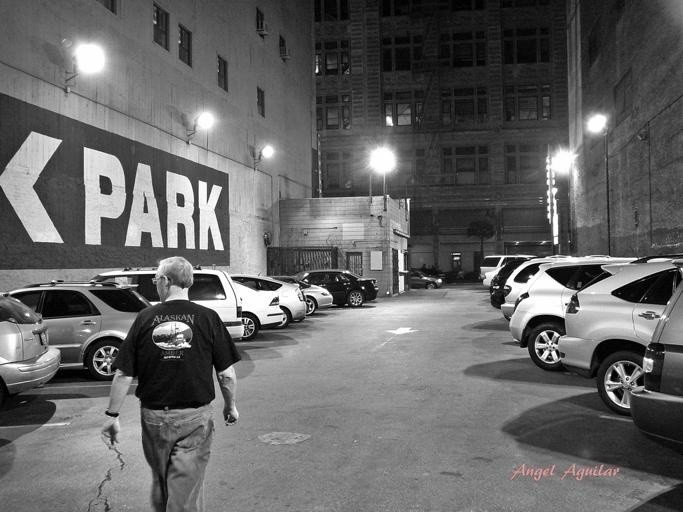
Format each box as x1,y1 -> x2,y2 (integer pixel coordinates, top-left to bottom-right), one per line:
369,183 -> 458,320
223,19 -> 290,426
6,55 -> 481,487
5,278 -> 153,380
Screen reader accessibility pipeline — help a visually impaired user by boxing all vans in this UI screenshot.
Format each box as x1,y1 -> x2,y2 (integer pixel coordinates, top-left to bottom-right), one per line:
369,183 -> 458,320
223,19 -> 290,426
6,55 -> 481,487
91,266 -> 245,340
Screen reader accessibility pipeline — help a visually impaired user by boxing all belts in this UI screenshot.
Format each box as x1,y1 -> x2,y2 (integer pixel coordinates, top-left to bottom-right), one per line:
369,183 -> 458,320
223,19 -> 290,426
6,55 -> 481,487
142,402 -> 207,410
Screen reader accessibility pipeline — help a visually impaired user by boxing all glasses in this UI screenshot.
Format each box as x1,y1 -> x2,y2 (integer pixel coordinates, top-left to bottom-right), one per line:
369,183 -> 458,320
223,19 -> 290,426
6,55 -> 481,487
151,276 -> 171,285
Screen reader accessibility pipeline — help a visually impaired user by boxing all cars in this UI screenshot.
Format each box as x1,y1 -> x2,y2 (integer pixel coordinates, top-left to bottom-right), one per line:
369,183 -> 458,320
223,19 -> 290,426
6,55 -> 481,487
558,253 -> 683,415
231,273 -> 334,341
630,279 -> 682,454
297,269 -> 377,307
410,267 -> 442,289
480,254 -> 556,321
509,256 -> 673,371
0,292 -> 62,411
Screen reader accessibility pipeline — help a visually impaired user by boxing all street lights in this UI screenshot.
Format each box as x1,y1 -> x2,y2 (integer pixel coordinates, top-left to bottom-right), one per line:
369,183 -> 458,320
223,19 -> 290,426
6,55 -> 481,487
370,145 -> 398,210
586,112 -> 611,257
551,148 -> 576,255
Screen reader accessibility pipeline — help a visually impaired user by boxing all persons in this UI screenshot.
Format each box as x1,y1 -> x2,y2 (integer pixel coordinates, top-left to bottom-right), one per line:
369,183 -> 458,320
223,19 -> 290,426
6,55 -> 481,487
421,262 -> 444,278
101,256 -> 243,512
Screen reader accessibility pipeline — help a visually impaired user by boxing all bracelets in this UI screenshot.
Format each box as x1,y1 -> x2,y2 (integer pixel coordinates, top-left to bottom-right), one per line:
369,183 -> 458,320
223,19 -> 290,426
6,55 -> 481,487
104,410 -> 120,418
